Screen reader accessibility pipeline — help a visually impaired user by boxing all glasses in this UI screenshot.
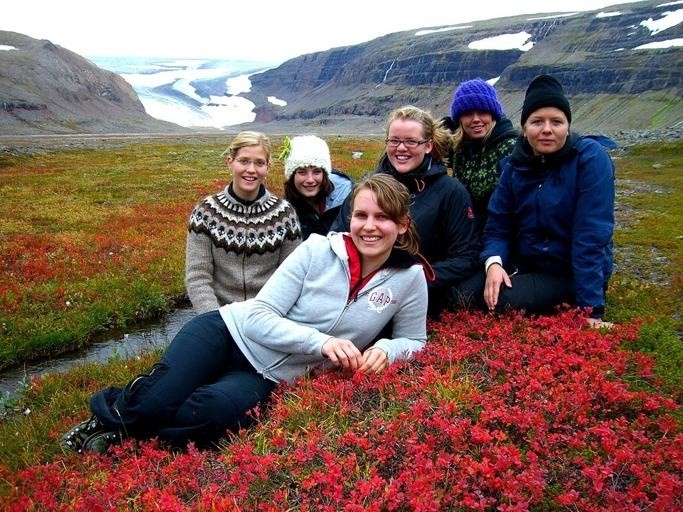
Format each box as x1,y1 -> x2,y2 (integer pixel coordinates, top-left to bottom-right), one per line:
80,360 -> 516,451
384,137 -> 429,148
232,158 -> 266,166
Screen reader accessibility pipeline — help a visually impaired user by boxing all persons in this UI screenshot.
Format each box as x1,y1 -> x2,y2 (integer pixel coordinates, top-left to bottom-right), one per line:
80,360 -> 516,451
283,133 -> 355,240
450,76 -> 623,334
328,103 -> 480,319
58,172 -> 436,458
181,129 -> 304,316
434,77 -> 519,233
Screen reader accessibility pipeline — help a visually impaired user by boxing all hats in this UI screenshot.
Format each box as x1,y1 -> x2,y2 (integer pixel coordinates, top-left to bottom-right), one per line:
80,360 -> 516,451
285,135 -> 331,179
451,78 -> 503,121
521,74 -> 571,126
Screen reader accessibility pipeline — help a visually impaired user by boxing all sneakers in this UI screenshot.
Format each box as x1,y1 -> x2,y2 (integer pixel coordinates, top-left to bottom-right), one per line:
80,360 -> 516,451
59,417 -> 121,453
